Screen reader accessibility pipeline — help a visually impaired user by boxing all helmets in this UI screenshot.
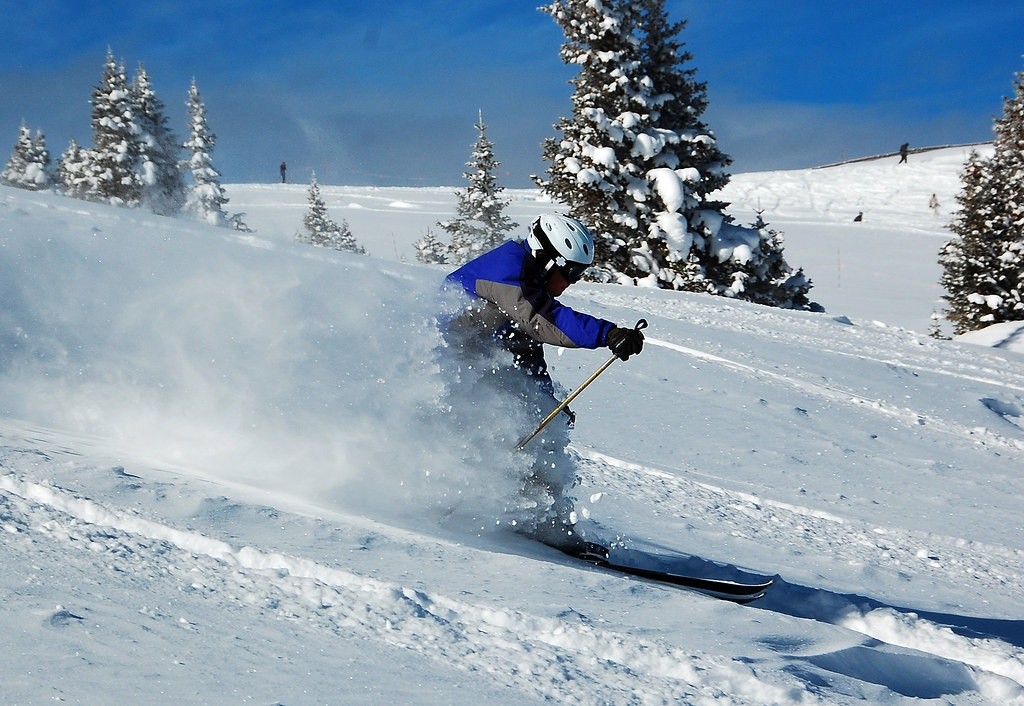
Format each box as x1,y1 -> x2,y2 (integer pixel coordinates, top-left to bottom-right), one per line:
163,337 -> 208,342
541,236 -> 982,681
527,214 -> 594,279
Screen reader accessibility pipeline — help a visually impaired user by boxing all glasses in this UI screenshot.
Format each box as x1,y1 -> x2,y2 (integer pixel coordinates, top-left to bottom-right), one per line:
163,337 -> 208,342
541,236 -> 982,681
532,218 -> 588,284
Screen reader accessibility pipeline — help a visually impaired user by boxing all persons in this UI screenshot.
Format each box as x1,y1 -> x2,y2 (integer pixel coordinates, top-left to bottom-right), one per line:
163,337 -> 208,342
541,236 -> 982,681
899,142 -> 909,164
432,211 -> 646,544
280,162 -> 287,183
854,212 -> 863,221
929,194 -> 938,208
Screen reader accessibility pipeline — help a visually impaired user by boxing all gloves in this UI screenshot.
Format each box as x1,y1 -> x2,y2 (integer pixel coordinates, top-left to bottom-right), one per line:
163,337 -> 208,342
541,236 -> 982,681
606,327 -> 643,361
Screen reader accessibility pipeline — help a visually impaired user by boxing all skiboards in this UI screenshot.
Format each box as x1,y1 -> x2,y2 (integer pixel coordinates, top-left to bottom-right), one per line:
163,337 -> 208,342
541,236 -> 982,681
555,543 -> 775,605
